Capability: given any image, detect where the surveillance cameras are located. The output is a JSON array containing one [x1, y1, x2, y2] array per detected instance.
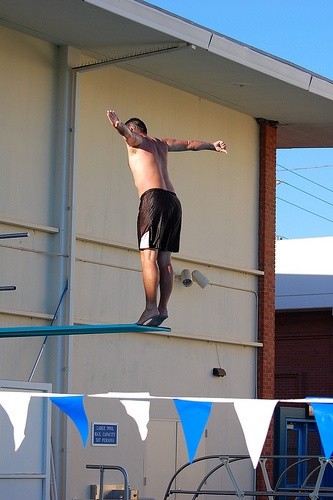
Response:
[[181, 268, 193, 288], [191, 269, 210, 289]]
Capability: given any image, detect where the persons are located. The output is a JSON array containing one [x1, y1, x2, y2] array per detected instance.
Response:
[[106, 110, 227, 327]]
